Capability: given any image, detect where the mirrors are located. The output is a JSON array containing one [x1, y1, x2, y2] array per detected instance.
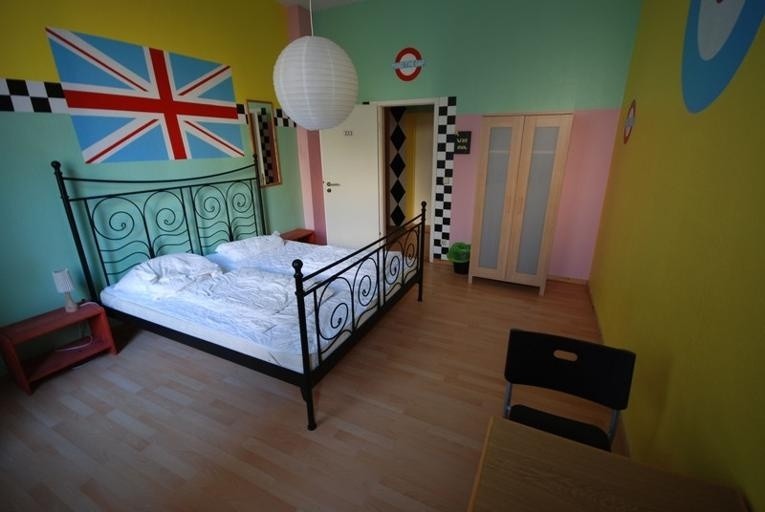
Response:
[[245, 98, 282, 188]]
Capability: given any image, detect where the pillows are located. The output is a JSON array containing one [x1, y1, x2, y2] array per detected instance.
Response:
[[215, 230, 285, 262], [113, 251, 225, 291]]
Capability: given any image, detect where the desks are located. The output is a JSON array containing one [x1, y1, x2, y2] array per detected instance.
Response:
[[280, 228, 315, 244], [2, 300, 120, 398], [464, 413, 751, 512]]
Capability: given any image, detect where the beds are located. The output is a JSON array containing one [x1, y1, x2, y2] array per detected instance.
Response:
[[51, 154, 427, 431]]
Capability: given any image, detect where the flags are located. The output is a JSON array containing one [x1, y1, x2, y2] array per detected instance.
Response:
[[42, 25, 246, 166]]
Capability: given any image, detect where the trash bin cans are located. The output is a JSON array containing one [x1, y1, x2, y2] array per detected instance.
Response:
[[452, 243, 470, 274]]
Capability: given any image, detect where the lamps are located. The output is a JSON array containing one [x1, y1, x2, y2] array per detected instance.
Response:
[[272, 1, 359, 132], [52, 268, 79, 312]]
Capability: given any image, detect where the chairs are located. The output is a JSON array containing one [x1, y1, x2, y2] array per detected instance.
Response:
[[502, 329, 636, 454]]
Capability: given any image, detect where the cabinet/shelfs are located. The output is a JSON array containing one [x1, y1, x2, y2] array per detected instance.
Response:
[[467, 110, 576, 298]]
